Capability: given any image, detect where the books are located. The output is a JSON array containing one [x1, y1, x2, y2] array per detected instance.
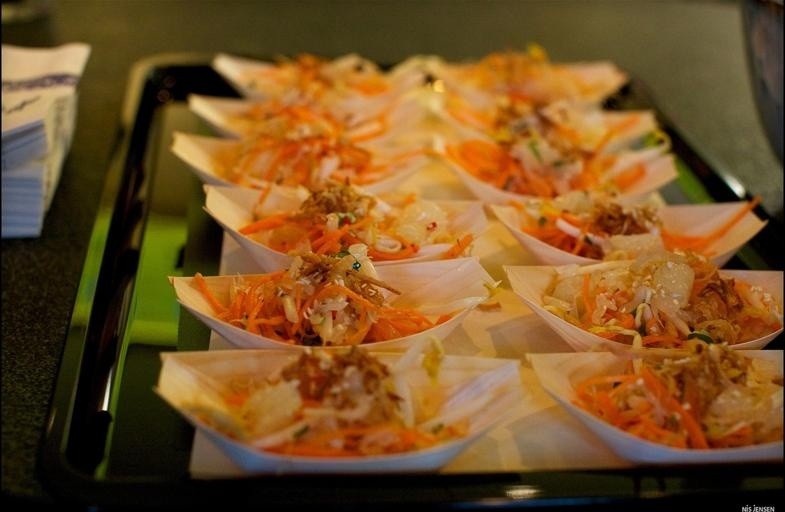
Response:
[[1, 41, 92, 239]]
